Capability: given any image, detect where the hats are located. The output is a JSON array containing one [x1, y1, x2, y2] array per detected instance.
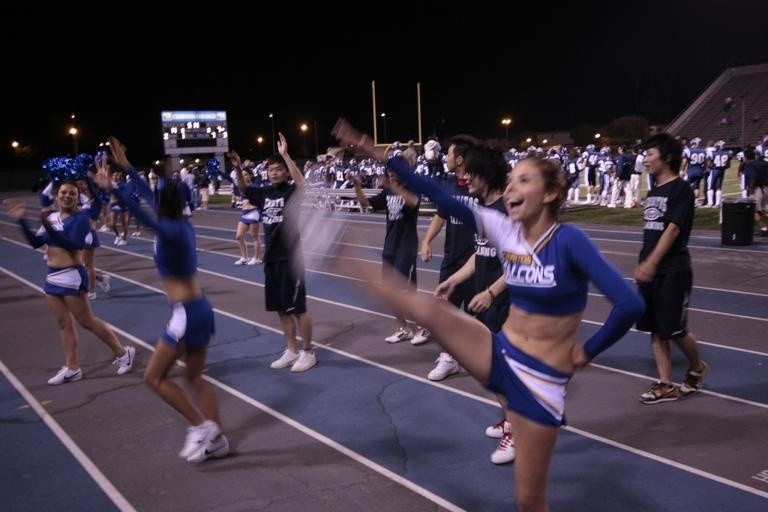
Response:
[[600, 146, 611, 155], [690, 137, 702, 145], [392, 141, 400, 150], [715, 141, 726, 149], [528, 145, 536, 155], [509, 148, 517, 157], [585, 144, 595, 151]]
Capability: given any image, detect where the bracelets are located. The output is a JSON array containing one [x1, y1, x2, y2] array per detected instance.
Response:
[[486, 286, 498, 300]]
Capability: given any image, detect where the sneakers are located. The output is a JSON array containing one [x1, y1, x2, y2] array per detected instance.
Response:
[[117, 240, 127, 246], [567, 197, 571, 201], [291, 351, 316, 372], [179, 420, 220, 458], [624, 203, 631, 208], [600, 199, 610, 206], [607, 203, 616, 208], [574, 199, 579, 205], [114, 237, 121, 245], [270, 349, 300, 369], [87, 292, 96, 301], [678, 360, 709, 399], [410, 326, 431, 344], [596, 198, 602, 204], [706, 201, 713, 208], [583, 200, 591, 205], [427, 352, 459, 380], [98, 275, 109, 293], [639, 381, 679, 404], [384, 328, 414, 344], [715, 203, 720, 208], [485, 420, 505, 438], [589, 200, 597, 204], [234, 257, 250, 265], [760, 230, 768, 237], [112, 346, 136, 375], [187, 435, 229, 463], [246, 257, 260, 265], [48, 366, 82, 385], [491, 421, 516, 464]]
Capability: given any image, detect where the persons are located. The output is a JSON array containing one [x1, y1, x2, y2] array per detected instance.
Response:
[[705, 141, 732, 209], [229, 131, 317, 374], [128, 213, 141, 238], [87, 138, 230, 464], [387, 140, 402, 158], [1, 182, 136, 385], [178, 163, 189, 181], [433, 143, 515, 465], [424, 134, 442, 178], [78, 176, 110, 302], [93, 152, 112, 234], [606, 145, 634, 210], [736, 153, 745, 202], [181, 169, 197, 211], [31, 174, 56, 261], [197, 174, 210, 209], [346, 148, 434, 347], [107, 173, 131, 247], [737, 148, 768, 236], [632, 132, 709, 405], [596, 146, 614, 207], [629, 147, 644, 206], [576, 143, 598, 205], [565, 151, 584, 204], [420, 134, 475, 381], [233, 168, 265, 267], [685, 139, 707, 208], [286, 118, 648, 512], [298, 155, 385, 188], [403, 136, 417, 170], [505, 146, 543, 167]]
